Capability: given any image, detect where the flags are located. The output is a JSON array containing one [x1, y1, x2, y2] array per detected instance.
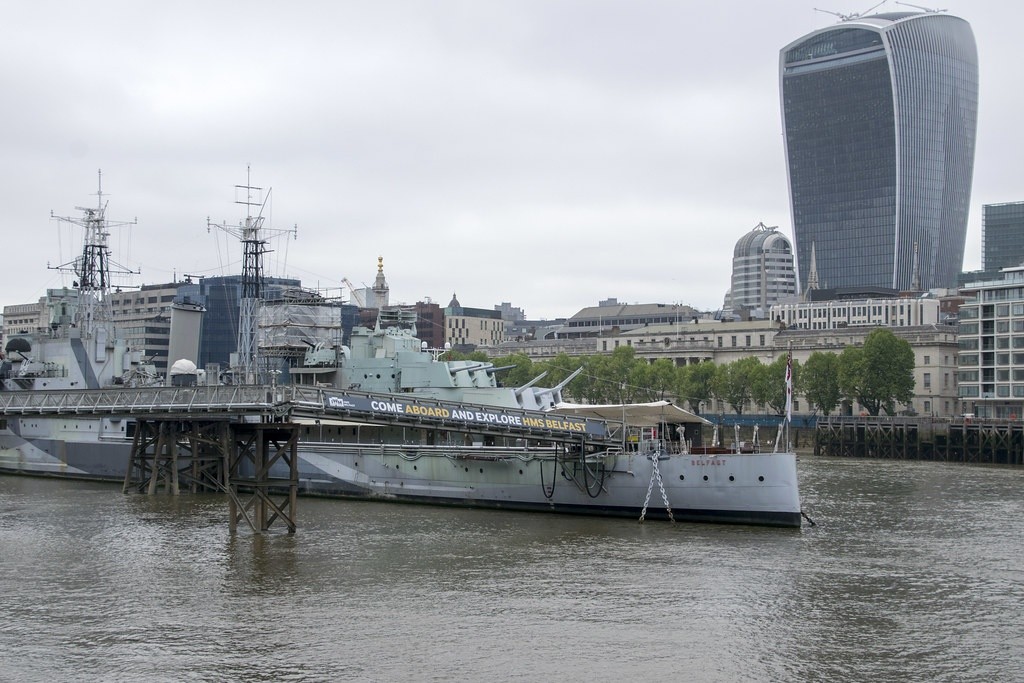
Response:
[[785, 350, 792, 423]]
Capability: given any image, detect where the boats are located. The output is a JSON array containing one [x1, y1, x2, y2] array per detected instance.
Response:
[[1, 156, 802, 530]]
